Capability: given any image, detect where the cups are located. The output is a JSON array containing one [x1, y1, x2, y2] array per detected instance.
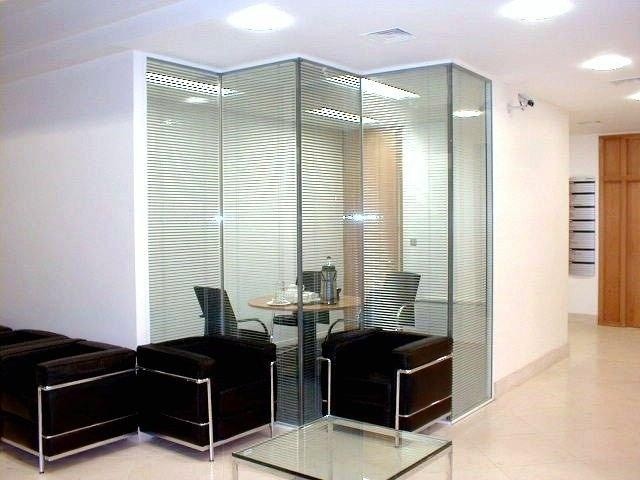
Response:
[[270, 280, 319, 304]]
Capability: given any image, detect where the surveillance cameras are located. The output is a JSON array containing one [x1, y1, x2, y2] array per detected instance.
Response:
[[517, 93, 534, 107]]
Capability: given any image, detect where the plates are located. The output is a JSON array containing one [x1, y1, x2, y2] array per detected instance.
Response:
[[266, 300, 292, 307]]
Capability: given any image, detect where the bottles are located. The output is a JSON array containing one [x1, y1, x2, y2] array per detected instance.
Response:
[[318, 266, 339, 304]]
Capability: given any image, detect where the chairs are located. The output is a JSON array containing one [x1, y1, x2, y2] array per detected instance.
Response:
[[193, 285, 270, 343], [137, 334, 278, 461], [1, 326, 137, 474], [327, 271, 421, 339], [319, 328, 452, 448]]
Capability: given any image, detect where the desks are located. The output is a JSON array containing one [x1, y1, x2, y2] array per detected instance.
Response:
[[247, 293, 363, 382]]
